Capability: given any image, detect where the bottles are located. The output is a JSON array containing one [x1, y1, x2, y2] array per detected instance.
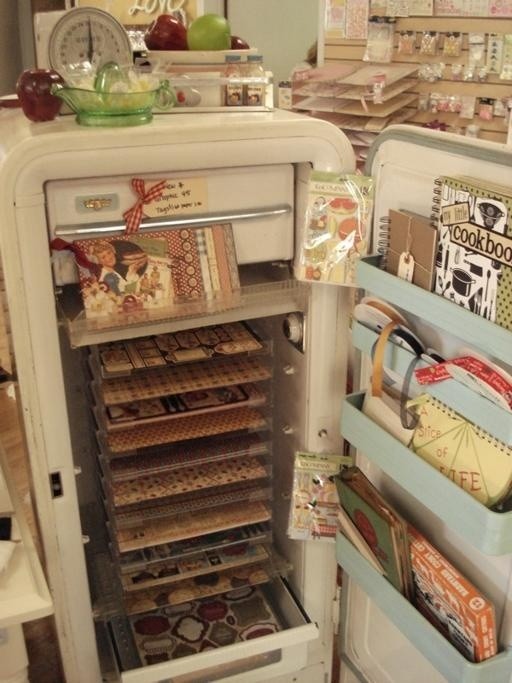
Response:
[[244, 55, 266, 107], [223, 55, 244, 106]]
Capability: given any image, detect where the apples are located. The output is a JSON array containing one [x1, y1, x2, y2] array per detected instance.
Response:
[[230, 36, 249, 50], [186, 14, 231, 50], [15, 69, 68, 123], [144, 13, 188, 51]]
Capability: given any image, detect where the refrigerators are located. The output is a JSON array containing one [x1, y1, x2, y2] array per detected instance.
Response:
[[0, 94, 512, 683]]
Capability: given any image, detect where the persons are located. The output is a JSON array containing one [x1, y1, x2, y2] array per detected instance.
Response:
[[89, 241, 162, 309]]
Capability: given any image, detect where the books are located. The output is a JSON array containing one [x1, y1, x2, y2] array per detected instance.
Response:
[[429, 174, 512, 331], [334, 464, 414, 605], [379, 207, 438, 294]]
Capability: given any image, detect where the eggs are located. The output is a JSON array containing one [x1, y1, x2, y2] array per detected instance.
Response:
[[175, 88, 203, 106]]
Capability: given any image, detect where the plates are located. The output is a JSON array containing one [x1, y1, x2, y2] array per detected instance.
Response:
[[146, 46, 259, 63]]
